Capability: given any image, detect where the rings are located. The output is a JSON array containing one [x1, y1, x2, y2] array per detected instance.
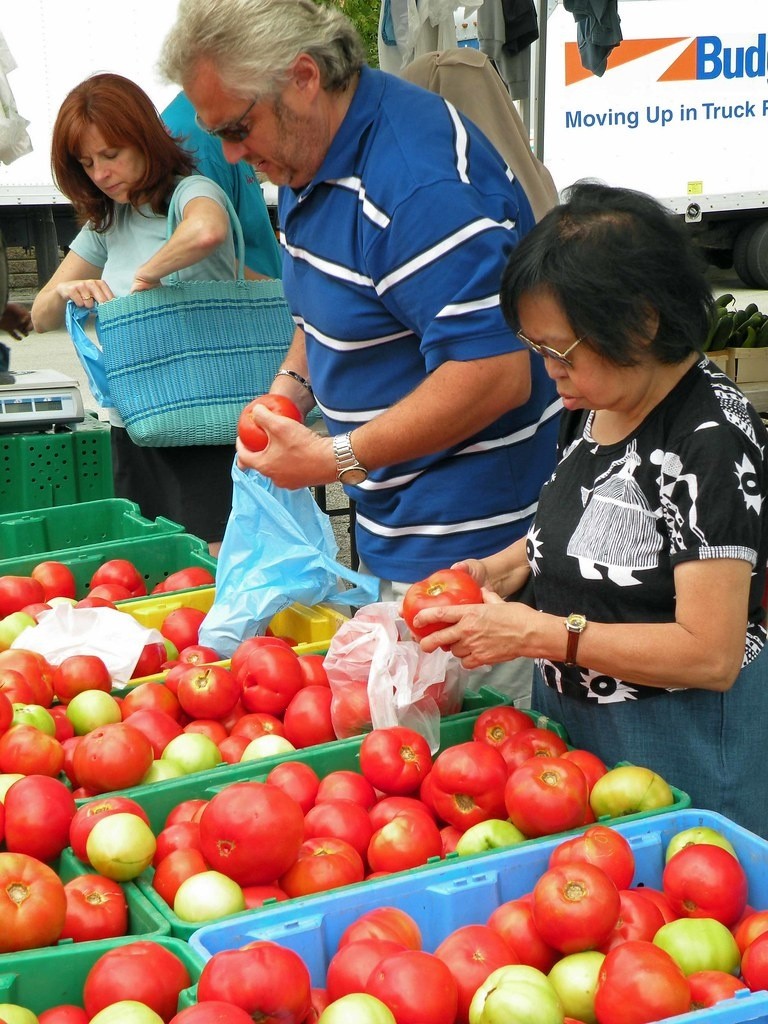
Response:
[[459, 659, 472, 671], [82, 295, 94, 301]]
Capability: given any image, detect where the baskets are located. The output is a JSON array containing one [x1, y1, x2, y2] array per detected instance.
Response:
[[0, 499, 768, 1023]]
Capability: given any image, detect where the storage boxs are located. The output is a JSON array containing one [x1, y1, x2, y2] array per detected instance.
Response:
[[0, 497, 768, 1024], [0, 409, 115, 514]]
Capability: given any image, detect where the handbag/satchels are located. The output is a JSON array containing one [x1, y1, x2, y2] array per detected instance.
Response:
[[324, 602, 491, 762], [198, 450, 379, 658], [97, 175, 323, 447]]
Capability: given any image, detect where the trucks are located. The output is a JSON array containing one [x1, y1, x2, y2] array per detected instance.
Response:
[[375, 0, 768, 291]]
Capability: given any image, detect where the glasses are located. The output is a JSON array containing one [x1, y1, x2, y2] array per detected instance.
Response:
[[513, 329, 593, 368], [196, 95, 259, 142]]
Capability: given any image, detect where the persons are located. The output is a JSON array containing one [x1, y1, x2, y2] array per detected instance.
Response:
[[399, 177, 767, 839], [0, 0, 566, 602]]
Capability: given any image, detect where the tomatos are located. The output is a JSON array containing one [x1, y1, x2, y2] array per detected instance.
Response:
[[0, 559, 768, 1024], [238, 394, 303, 452]]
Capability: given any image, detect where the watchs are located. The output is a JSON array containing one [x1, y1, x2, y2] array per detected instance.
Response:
[[333, 430, 368, 485], [563, 612, 587, 667]]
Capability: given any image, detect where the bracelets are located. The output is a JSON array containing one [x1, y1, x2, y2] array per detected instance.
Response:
[[274, 370, 317, 407]]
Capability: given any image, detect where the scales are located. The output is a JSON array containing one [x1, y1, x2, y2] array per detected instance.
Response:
[[0, 368, 86, 429]]
